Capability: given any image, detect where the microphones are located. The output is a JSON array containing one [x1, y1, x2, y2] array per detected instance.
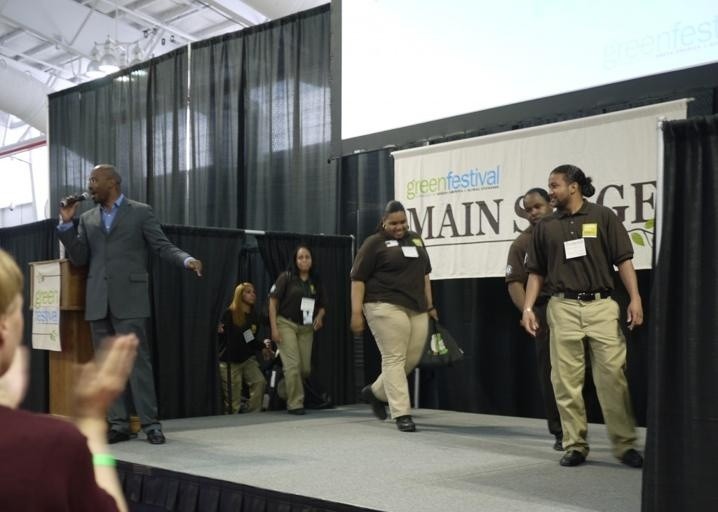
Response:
[[58, 192, 91, 207]]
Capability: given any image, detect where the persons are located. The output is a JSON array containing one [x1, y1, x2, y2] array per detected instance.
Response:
[[505, 188, 567, 451], [349, 200, 439, 432], [521, 164, 644, 469], [267, 243, 327, 415], [216, 282, 265, 413], [56, 164, 202, 445], [0, 249, 139, 511], [261, 338, 277, 360]]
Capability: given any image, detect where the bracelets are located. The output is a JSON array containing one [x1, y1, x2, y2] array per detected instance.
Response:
[[93, 452, 118, 469], [522, 307, 535, 313], [428, 306, 435, 313]]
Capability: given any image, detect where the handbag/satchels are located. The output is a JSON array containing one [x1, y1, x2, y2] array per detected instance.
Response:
[[426, 317, 464, 369]]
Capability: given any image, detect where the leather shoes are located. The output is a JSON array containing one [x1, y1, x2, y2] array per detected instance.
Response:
[[620, 448, 642, 468], [397, 416, 416, 432], [107, 430, 128, 443], [559, 451, 585, 466], [554, 288, 614, 302], [147, 429, 166, 444], [362, 385, 388, 419], [289, 407, 306, 415], [553, 437, 563, 451]]
